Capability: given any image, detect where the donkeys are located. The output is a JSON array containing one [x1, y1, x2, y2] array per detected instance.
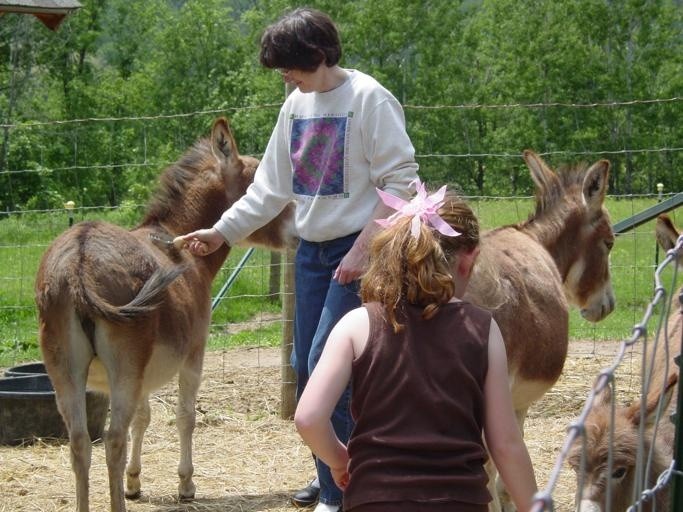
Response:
[[566, 212, 682, 512], [32, 118, 301, 512], [453, 149, 616, 512]]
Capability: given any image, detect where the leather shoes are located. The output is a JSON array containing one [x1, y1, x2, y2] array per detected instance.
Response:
[[291, 477, 320, 505]]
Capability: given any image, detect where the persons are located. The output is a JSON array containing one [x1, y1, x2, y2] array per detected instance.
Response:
[[183, 9, 420, 512], [293, 176, 538, 512]]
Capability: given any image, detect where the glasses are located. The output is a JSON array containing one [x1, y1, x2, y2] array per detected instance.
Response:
[[273, 68, 296, 79]]
